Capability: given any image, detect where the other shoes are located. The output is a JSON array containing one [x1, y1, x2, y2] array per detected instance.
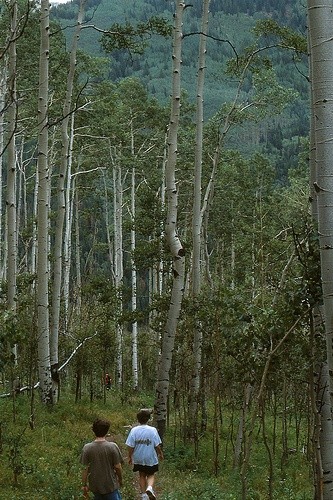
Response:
[[142, 496, 149, 500], [145, 488, 157, 500]]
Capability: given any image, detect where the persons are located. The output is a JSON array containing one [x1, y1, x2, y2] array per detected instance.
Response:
[[79, 420, 125, 500], [104, 374, 112, 390], [125, 409, 164, 500]]
